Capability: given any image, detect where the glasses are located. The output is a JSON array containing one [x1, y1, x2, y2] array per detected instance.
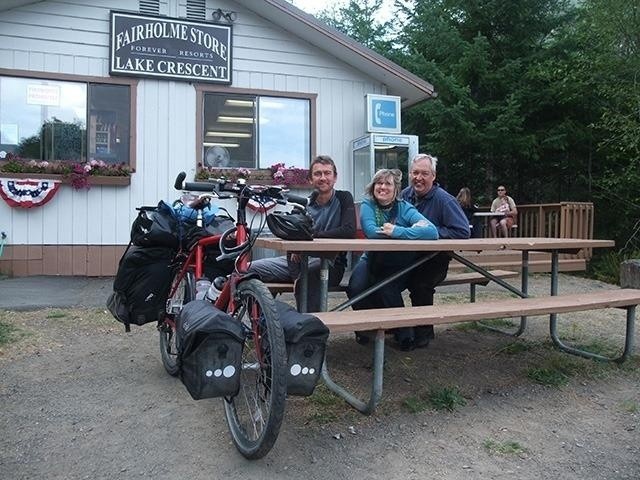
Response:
[[497, 189, 505, 191]]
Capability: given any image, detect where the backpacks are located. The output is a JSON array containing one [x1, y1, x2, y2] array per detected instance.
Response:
[[107, 207, 237, 332]]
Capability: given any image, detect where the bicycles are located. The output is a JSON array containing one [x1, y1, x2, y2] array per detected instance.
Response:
[[105, 171, 328, 460]]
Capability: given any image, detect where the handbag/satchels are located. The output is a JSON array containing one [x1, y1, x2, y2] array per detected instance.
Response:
[[175, 300, 245, 400], [261, 299, 330, 397]]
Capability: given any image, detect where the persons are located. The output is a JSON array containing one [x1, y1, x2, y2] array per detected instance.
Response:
[[347, 153, 469, 349], [455, 186, 481, 238], [490, 184, 519, 238], [246, 156, 356, 313], [358, 168, 438, 347]]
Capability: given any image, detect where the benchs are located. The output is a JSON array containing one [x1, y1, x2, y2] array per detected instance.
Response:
[[469, 212, 518, 238], [254, 268, 514, 337], [300, 285, 640, 406]]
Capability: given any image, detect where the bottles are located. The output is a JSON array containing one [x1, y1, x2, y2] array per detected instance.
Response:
[[201, 276, 227, 303]]
